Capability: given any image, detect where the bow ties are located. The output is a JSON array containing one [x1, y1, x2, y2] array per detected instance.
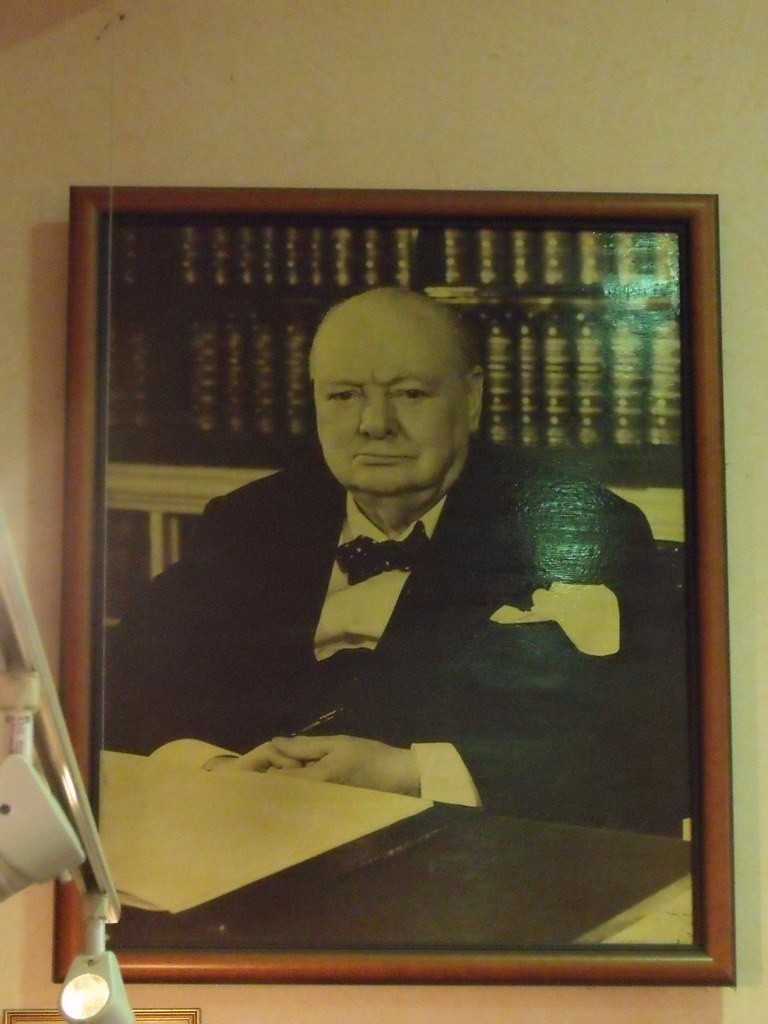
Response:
[[335, 519, 430, 586]]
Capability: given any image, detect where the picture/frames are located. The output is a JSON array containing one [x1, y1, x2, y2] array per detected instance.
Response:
[[47, 185, 739, 989]]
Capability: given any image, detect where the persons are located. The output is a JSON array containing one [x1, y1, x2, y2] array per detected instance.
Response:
[[102, 288, 693, 835]]
